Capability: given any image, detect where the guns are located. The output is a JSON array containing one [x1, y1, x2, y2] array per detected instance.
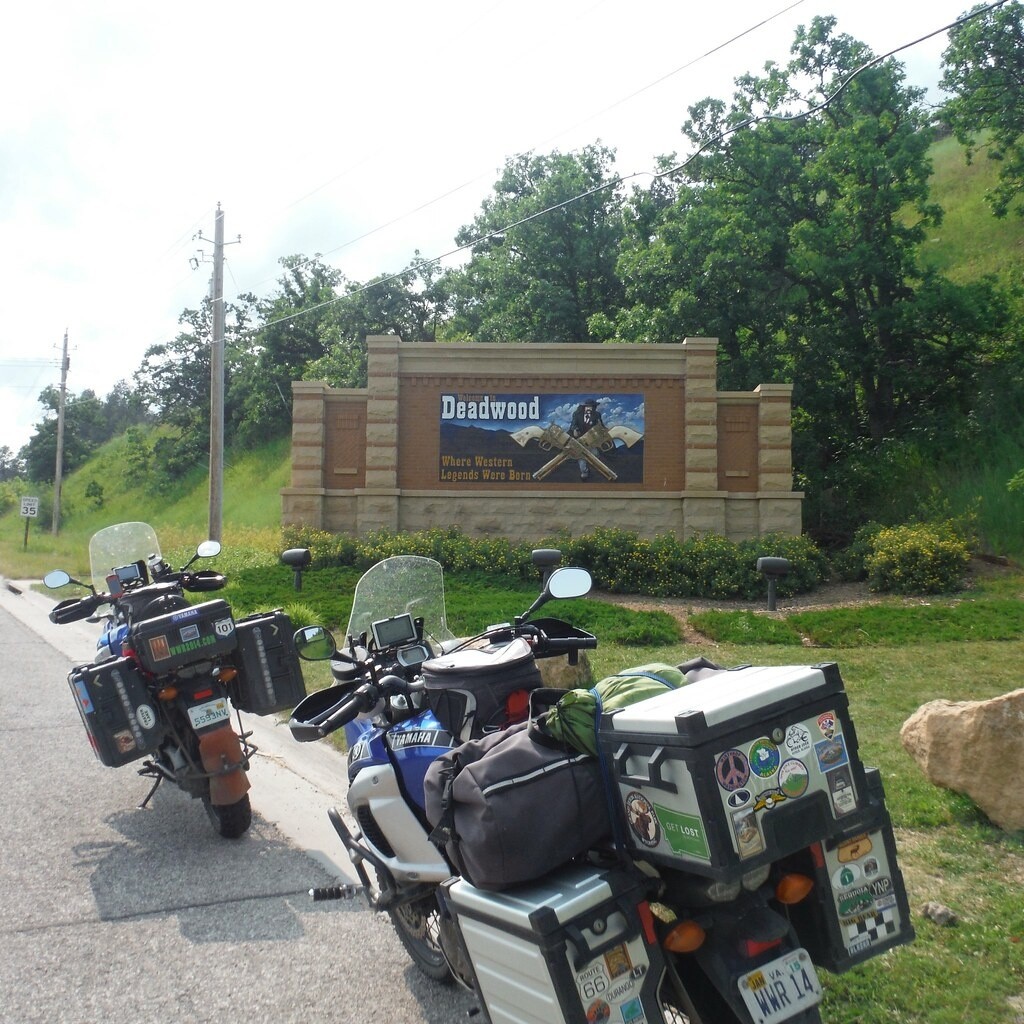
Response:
[[532, 425, 643, 481], [510, 424, 617, 481]]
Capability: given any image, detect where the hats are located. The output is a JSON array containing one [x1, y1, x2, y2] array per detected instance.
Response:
[[583, 398, 600, 407]]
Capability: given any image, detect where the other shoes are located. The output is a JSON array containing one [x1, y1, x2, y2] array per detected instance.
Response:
[[580, 472, 589, 479]]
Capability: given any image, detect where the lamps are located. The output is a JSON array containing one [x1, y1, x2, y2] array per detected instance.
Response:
[[757, 557, 789, 610], [282, 549, 311, 592], [532, 550, 561, 591]]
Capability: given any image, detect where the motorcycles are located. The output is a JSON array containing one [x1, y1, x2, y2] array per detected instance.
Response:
[[287, 555, 917, 1024], [41, 521, 310, 842]]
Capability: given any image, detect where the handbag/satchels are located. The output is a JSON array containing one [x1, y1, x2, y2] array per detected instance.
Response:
[[423, 655, 727, 890]]
